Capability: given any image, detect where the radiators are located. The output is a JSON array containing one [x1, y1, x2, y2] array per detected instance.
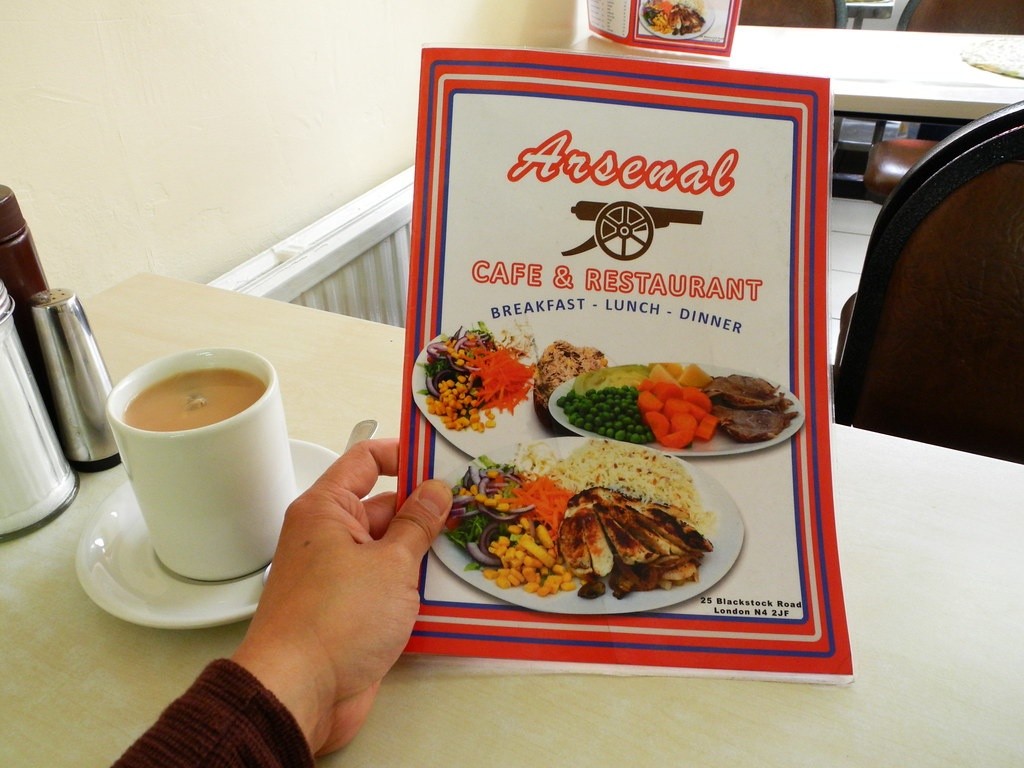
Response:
[[205, 163, 415, 327]]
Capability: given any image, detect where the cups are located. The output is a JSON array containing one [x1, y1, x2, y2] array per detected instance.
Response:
[[105, 348, 298, 581]]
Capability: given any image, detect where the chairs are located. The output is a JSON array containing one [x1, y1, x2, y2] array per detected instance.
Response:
[[736, 0, 1024, 464]]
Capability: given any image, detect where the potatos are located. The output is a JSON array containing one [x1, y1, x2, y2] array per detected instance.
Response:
[[647, 362, 714, 389]]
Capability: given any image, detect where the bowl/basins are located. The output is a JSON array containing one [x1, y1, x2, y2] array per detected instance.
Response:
[[533, 386, 581, 437]]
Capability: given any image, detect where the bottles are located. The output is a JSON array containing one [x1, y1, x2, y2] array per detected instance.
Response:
[[0, 183, 90, 437], [31, 288, 121, 473]]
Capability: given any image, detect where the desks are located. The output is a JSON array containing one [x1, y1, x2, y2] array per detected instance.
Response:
[[1, 273, 1024, 768], [568, 24, 1024, 160]]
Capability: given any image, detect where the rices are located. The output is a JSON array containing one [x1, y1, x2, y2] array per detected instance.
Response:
[[544, 439, 716, 537]]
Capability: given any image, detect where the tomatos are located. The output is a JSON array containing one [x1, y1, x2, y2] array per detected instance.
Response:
[[573, 365, 652, 395]]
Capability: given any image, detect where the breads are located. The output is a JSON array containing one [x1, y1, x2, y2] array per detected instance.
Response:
[[559, 486, 716, 580]]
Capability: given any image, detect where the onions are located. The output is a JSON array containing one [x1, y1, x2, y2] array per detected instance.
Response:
[[425, 326, 490, 398]]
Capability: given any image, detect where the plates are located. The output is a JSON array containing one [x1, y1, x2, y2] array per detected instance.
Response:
[[75, 440, 368, 630], [549, 363, 804, 455], [639, 0, 715, 39], [430, 437, 745, 614], [412, 319, 618, 460]]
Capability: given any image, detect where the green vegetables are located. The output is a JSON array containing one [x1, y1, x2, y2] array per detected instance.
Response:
[[444, 454, 520, 571]]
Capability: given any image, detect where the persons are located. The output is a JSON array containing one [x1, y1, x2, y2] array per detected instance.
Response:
[[109, 440, 454, 768]]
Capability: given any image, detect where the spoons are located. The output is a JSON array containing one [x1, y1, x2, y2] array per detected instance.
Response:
[[262, 421, 377, 585]]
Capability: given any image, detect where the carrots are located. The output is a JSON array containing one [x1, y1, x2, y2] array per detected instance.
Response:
[[636, 380, 718, 447], [500, 475, 575, 542], [463, 343, 539, 416]]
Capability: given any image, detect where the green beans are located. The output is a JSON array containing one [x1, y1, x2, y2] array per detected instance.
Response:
[[555, 383, 654, 443]]
